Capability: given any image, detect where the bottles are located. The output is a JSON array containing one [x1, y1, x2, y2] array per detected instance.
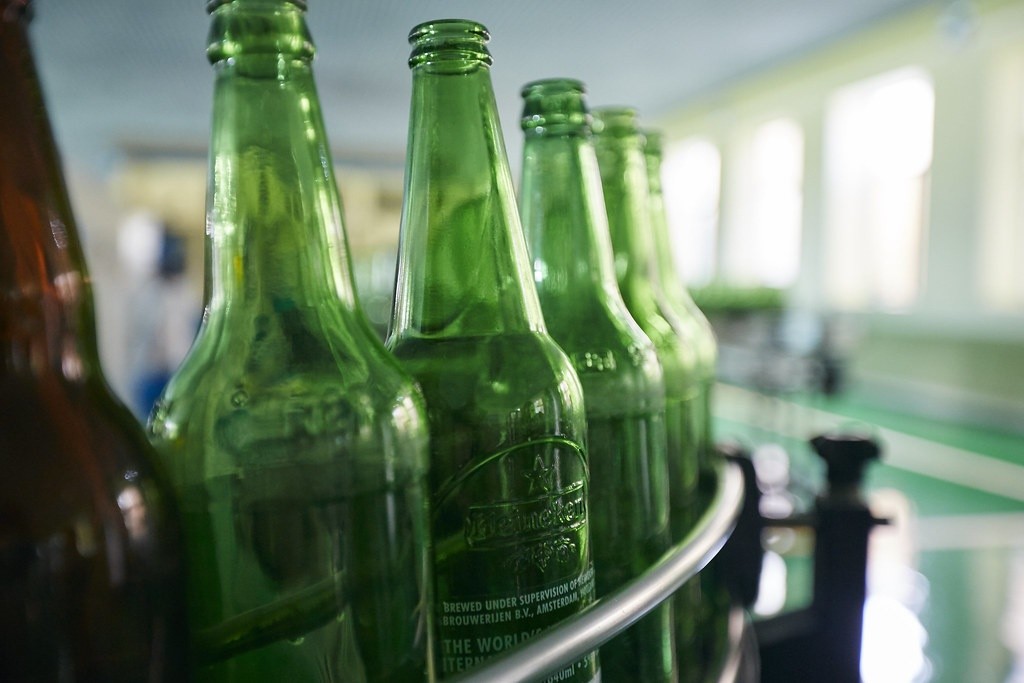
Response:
[[517, 78, 672, 683], [145, 1, 433, 683], [589, 105, 719, 683], [0, 0, 181, 683], [384, 19, 602, 683]]
